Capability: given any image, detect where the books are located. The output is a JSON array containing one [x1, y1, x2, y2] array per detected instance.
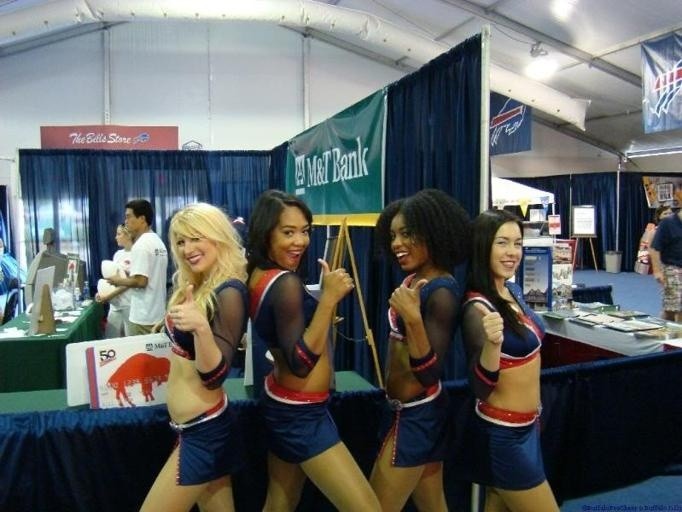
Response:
[[545, 298, 682, 344]]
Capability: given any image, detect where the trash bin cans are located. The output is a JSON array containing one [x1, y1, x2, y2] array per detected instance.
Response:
[[605, 250, 623, 273]]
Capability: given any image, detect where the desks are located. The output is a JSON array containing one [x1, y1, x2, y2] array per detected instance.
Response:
[[0, 371, 378, 415], [0, 297, 107, 393], [534, 301, 682, 369]]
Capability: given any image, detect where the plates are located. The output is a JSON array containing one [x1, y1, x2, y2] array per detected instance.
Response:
[[554, 302, 663, 332]]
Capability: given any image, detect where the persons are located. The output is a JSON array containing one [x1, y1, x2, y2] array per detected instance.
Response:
[[135, 200, 250, 512], [636, 201, 674, 277], [106, 197, 169, 335], [649, 181, 681, 325], [456, 208, 562, 512], [93, 221, 136, 340], [246, 184, 385, 512], [366, 185, 478, 511]]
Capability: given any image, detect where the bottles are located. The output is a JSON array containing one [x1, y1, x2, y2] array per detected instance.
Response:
[[83, 280, 90, 302], [73, 288, 82, 311]]
